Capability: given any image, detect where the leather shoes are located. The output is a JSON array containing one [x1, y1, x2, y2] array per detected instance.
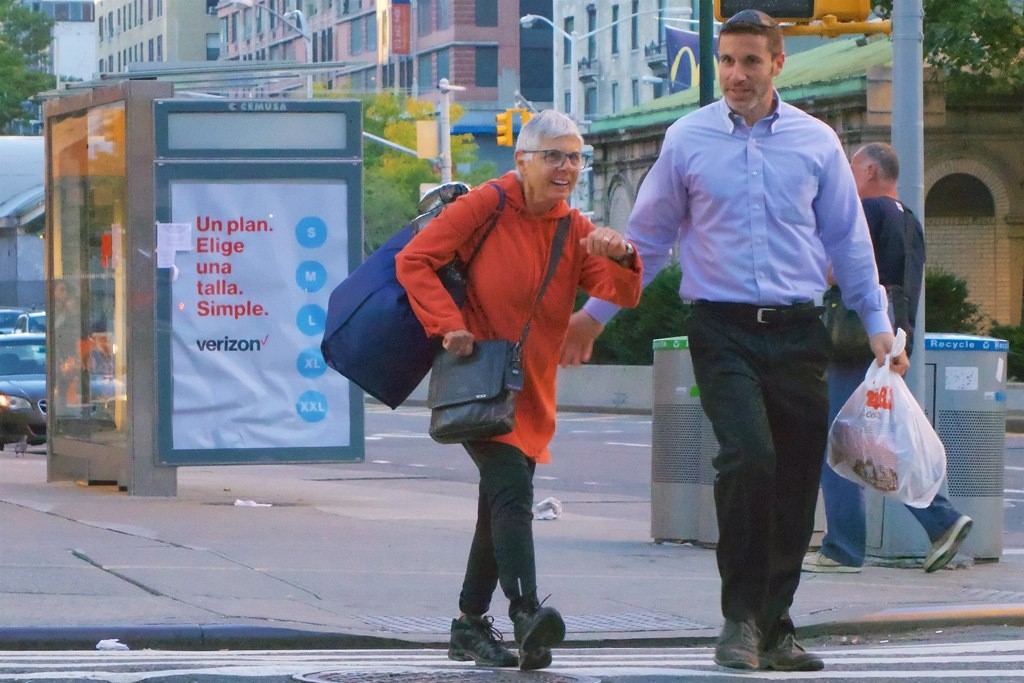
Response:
[[758, 629, 825, 672], [714, 614, 760, 671], [691, 298, 826, 327]]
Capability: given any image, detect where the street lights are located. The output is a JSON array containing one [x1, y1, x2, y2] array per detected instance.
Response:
[[232, 0, 312, 101], [520, 5, 695, 221]]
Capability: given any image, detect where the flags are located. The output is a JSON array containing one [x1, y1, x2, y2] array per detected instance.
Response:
[[665, 25, 721, 92]]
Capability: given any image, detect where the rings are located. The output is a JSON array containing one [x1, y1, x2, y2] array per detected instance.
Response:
[[604, 237, 609, 242]]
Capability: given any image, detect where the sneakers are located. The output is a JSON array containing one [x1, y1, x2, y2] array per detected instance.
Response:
[[448, 614, 520, 668], [513, 594, 566, 670], [801, 551, 862, 574], [922, 514, 974, 573]]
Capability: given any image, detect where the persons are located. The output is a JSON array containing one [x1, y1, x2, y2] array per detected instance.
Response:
[[394, 107, 643, 671], [53, 280, 81, 409], [801, 142, 974, 574], [558, 10, 910, 670]]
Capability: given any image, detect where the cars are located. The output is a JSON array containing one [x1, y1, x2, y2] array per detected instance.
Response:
[[0, 305, 120, 452]]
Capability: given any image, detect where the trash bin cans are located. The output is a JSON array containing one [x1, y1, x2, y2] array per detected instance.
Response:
[[861, 332, 1009, 563], [650, 338, 719, 549]]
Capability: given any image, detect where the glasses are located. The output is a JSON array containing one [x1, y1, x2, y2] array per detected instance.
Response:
[[523, 149, 592, 171]]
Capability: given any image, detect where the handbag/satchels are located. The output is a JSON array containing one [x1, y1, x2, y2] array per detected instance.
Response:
[[825, 327, 946, 509], [320, 180, 509, 409], [823, 287, 916, 367], [426, 336, 521, 443]]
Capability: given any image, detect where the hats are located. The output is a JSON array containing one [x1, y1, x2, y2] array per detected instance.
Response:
[[725, 9, 778, 28]]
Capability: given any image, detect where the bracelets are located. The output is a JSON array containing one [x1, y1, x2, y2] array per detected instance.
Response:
[[625, 242, 634, 255]]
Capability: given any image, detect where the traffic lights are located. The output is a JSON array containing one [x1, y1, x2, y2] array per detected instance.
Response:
[[714, 0, 872, 23]]
